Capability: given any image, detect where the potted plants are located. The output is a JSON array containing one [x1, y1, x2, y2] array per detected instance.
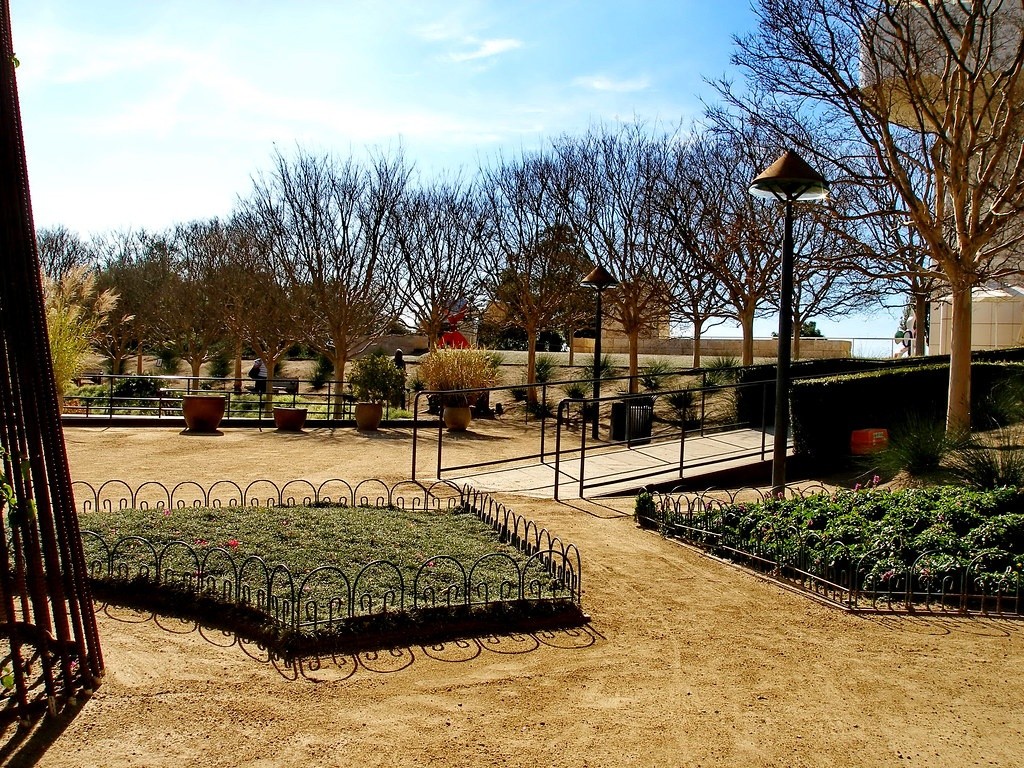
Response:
[[346, 353, 407, 431], [418, 338, 501, 433]]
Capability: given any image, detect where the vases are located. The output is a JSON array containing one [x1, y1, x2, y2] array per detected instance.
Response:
[[181, 394, 227, 434], [273, 407, 308, 432]]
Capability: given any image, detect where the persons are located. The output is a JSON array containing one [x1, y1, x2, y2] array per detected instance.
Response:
[[253, 351, 269, 392], [389, 349, 406, 410]]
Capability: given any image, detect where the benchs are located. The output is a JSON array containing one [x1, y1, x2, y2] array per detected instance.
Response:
[[71, 367, 104, 386], [271, 376, 300, 394]]
